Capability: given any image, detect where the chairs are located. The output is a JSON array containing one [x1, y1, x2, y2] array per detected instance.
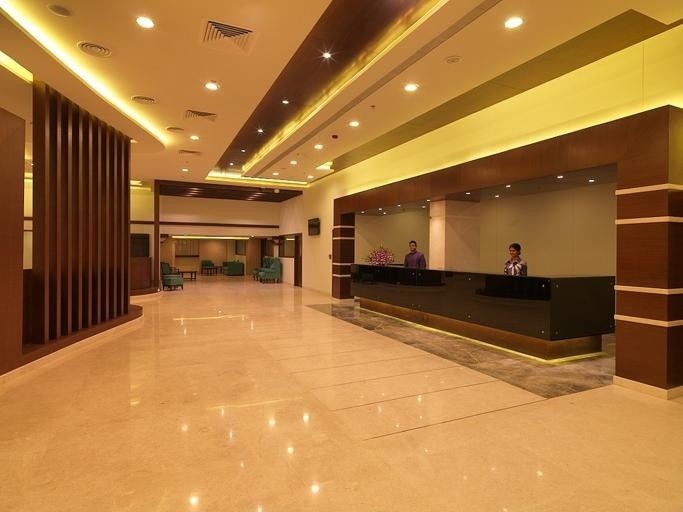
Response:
[[160, 255, 282, 291]]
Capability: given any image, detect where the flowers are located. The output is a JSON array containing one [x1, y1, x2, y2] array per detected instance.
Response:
[[360, 240, 395, 266]]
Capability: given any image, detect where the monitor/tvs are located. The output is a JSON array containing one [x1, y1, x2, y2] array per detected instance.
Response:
[[307, 218, 320, 236]]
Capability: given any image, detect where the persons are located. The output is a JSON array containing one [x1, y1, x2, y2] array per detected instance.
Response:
[[404, 240, 426, 269], [504, 242, 527, 277]]
